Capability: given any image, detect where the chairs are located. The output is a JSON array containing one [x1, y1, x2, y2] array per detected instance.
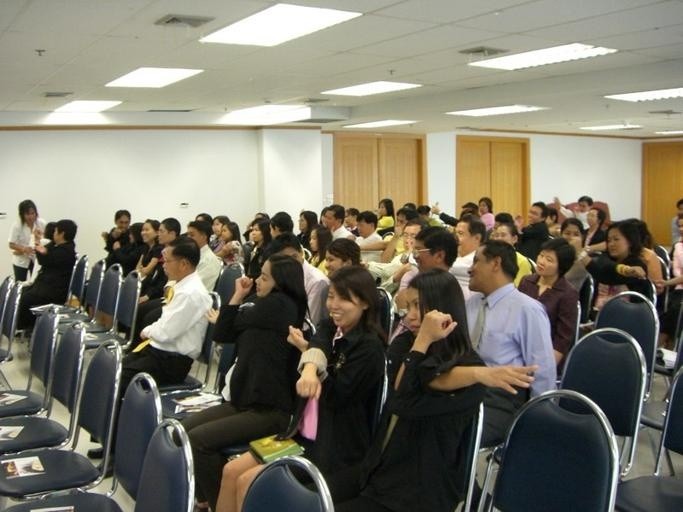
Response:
[[0, 248, 317, 512], [241, 455, 335, 511], [377, 241, 683, 512]]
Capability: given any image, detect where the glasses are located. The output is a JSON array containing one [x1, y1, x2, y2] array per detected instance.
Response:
[[410, 247, 431, 257]]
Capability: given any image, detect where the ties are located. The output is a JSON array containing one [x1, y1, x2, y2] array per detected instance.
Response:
[[131, 287, 173, 353], [471, 298, 488, 349]]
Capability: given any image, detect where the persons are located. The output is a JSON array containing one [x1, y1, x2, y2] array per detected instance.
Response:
[[459, 240, 556, 510], [78, 238, 210, 458], [213, 266, 387, 512], [170, 254, 306, 504], [306, 270, 538, 511]]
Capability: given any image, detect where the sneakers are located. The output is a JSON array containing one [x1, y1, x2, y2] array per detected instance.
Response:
[[95, 462, 114, 477], [87, 446, 104, 458]]
